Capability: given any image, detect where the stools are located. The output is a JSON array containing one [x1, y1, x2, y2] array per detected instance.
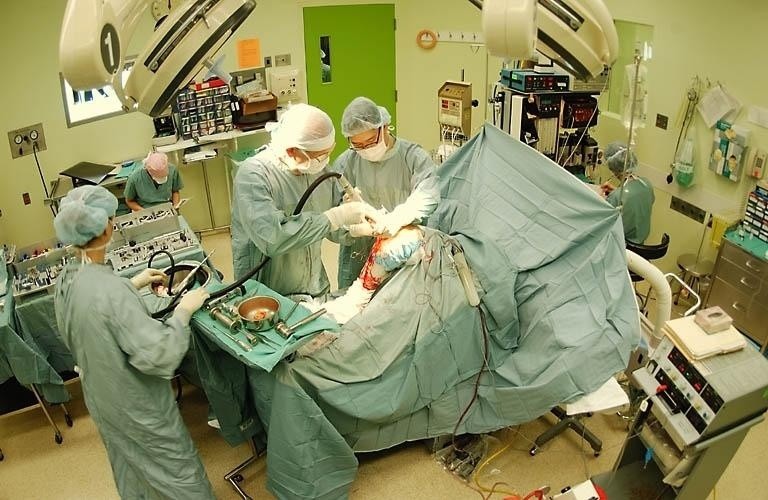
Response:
[[530, 375, 630, 458], [669, 252, 715, 305]]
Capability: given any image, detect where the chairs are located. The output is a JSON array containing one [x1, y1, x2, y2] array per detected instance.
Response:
[[624, 233, 672, 294]]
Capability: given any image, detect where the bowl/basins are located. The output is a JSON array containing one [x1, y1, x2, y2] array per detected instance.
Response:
[[237, 294, 281, 331]]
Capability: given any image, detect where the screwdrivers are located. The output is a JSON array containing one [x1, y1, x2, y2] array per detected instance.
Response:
[[212, 324, 253, 354]]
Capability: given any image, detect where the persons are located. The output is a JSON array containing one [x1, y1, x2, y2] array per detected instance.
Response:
[[124, 152, 183, 213], [219, 105, 362, 299], [601, 143, 655, 243], [52, 187, 214, 500], [298, 220, 425, 326], [329, 97, 438, 290]]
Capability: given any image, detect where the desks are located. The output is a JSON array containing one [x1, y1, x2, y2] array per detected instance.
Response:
[[44, 156, 145, 217], [151, 124, 270, 244], [0, 249, 72, 460], [13, 215, 223, 428]]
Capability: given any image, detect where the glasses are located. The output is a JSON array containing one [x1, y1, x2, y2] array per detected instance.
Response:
[[307, 143, 338, 164], [346, 128, 382, 151]]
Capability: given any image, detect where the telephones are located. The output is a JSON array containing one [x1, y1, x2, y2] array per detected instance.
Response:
[[745, 148, 766, 179]]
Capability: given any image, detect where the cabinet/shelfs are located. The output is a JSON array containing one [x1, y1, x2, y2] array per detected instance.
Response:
[[702, 238, 768, 355]]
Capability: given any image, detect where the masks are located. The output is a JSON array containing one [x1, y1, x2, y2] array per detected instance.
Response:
[[148, 171, 168, 185], [350, 125, 391, 162], [284, 147, 330, 175]]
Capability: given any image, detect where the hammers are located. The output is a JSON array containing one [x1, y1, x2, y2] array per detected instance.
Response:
[[275, 308, 327, 339]]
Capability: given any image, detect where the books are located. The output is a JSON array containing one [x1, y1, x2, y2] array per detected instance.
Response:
[[664, 317, 746, 362]]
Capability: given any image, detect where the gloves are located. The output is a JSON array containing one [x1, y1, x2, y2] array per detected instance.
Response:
[[129, 267, 168, 290], [180, 286, 211, 315], [323, 186, 409, 239]]
